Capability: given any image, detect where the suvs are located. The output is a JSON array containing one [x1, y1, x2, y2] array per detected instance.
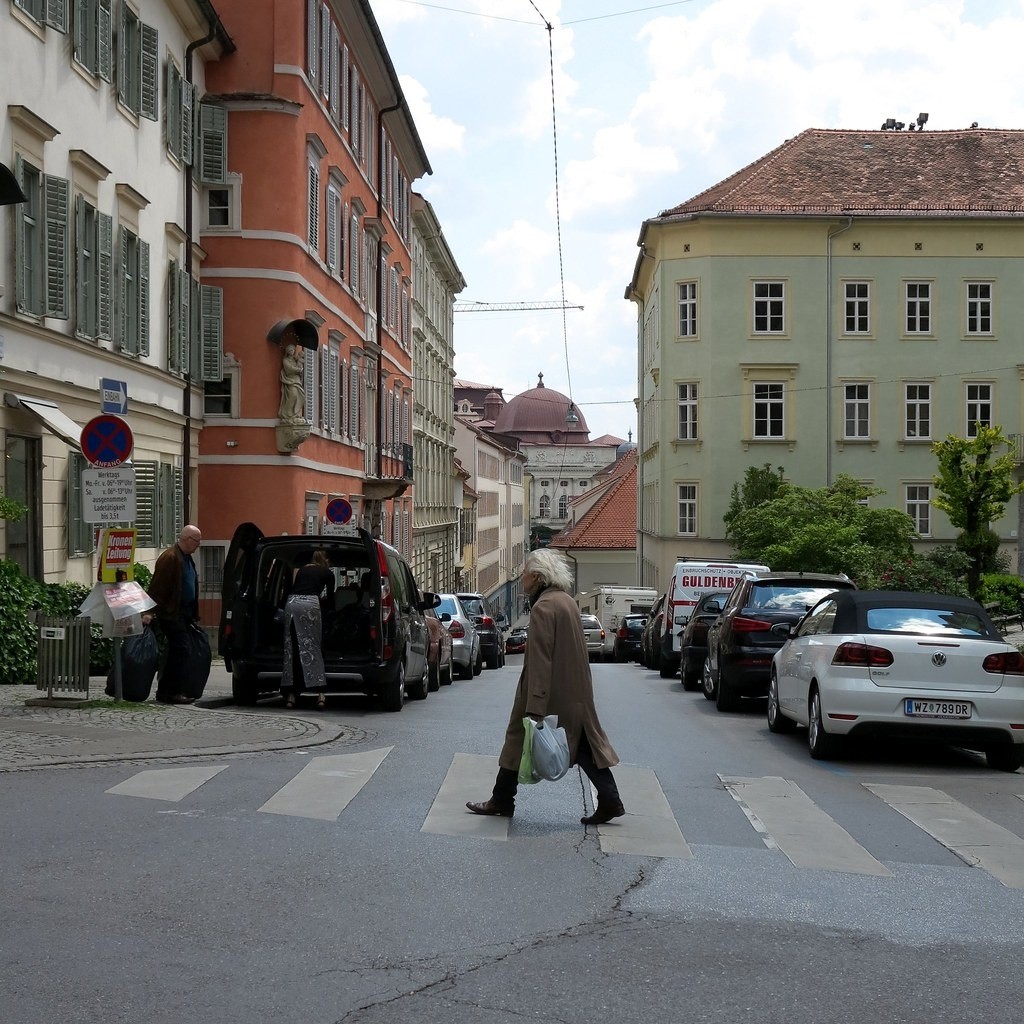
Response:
[[456, 591, 511, 670], [700, 568, 860, 712]]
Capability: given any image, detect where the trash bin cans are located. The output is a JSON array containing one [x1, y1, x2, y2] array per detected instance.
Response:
[[25, 616, 91, 708]]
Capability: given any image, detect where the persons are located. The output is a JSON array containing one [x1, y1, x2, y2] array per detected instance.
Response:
[[281, 550, 335, 711], [141, 524, 203, 703], [464, 550, 626, 825]]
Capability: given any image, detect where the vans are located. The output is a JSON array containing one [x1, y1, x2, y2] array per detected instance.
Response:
[[659, 554, 771, 678], [217, 523, 444, 712]]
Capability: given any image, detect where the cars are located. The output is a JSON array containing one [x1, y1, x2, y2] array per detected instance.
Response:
[[418, 589, 455, 692], [578, 613, 606, 662], [504, 635, 526, 654], [764, 589, 1024, 773], [434, 594, 484, 680], [510, 625, 528, 640], [610, 613, 649, 663], [639, 593, 667, 670], [674, 587, 735, 692]]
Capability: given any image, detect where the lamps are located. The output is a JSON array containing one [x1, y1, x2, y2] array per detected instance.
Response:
[[565, 403, 579, 427]]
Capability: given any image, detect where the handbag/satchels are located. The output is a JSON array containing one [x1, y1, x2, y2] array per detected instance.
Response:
[[176, 619, 212, 699], [518, 715, 569, 785], [105, 624, 157, 702]]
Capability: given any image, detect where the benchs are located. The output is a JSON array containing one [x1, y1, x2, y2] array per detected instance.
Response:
[[984, 600, 1024, 636]]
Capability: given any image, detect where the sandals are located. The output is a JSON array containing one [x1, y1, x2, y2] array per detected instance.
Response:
[[285, 701, 296, 709], [316, 701, 326, 710]]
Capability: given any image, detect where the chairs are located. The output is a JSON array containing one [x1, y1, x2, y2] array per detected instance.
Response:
[[335, 587, 358, 611], [361, 572, 373, 591]]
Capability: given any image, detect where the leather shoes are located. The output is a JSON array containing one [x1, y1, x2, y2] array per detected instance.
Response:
[[466, 798, 515, 818], [580, 804, 625, 825], [156, 690, 195, 704]]
